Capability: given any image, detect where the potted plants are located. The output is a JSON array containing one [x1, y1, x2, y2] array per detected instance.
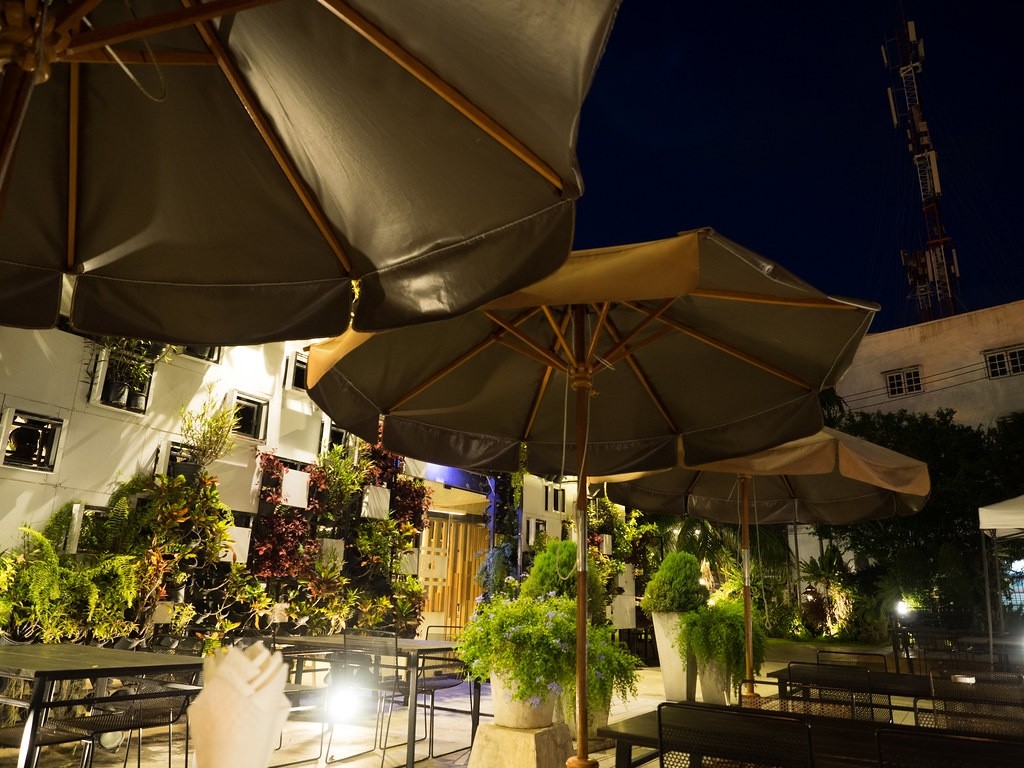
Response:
[[661, 603, 768, 706], [78, 334, 188, 412], [516, 538, 616, 739], [639, 548, 710, 702], [0, 382, 436, 680]]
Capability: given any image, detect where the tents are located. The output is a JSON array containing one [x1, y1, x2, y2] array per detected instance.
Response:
[[977, 489, 1024, 677]]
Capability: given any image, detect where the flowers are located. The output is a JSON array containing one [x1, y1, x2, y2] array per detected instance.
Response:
[[450, 576, 648, 725]]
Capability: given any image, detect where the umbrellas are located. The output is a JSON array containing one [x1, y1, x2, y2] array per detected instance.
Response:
[[2, 1, 622, 349], [302, 228, 883, 759], [588, 425, 932, 696]]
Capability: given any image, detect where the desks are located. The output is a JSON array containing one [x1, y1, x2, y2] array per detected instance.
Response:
[[767, 664, 1024, 737], [0, 643, 207, 768], [244, 635, 482, 768], [595, 699, 1024, 768], [887, 623, 1024, 687]]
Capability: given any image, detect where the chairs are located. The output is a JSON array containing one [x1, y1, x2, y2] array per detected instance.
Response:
[[43, 633, 207, 768], [913, 694, 1024, 739], [812, 650, 892, 723], [737, 679, 857, 720], [928, 665, 1024, 736], [375, 626, 473, 759], [325, 628, 433, 768], [657, 702, 818, 768], [231, 628, 329, 768], [787, 662, 893, 723], [873, 728, 1024, 768], [0, 723, 96, 768]]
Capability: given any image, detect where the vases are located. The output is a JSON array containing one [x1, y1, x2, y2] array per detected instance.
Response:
[[188, 347, 212, 358], [488, 667, 557, 731]]
